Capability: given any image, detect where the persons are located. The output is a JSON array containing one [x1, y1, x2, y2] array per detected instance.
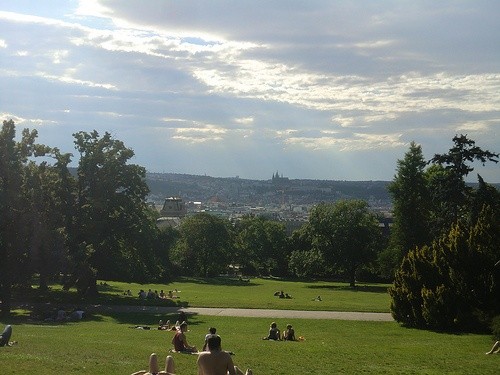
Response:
[[197, 335, 252, 375], [138, 289, 173, 301], [203, 327, 222, 351], [229, 280, 234, 286], [131, 353, 175, 375], [315, 296, 322, 301], [268, 322, 281, 341], [240, 277, 243, 281], [170, 321, 191, 332], [274, 291, 292, 299], [128, 290, 132, 296], [282, 324, 295, 341], [57, 307, 66, 321], [248, 278, 251, 282], [100, 282, 110, 288], [172, 324, 198, 353], [0, 325, 13, 347], [158, 323, 169, 330], [485, 341, 500, 354], [75, 308, 87, 320]]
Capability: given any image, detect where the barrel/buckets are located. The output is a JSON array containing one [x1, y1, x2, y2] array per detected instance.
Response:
[[76, 311, 84, 320]]
